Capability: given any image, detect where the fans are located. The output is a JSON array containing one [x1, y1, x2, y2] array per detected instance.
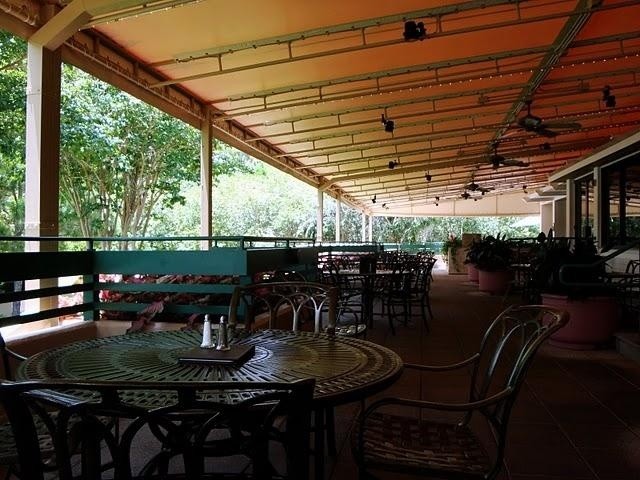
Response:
[[446, 96, 583, 201]]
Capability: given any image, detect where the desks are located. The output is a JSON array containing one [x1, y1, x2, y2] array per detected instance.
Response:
[[22, 323, 398, 418]]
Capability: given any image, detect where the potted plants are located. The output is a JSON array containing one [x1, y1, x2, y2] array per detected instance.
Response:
[[529, 234, 620, 350], [474, 235, 512, 294], [463, 241, 479, 281]]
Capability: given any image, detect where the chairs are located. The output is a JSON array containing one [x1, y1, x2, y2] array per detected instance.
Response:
[[351, 305, 568, 479], [317, 236, 438, 334], [229, 280, 333, 331], [5, 375, 315, 480]]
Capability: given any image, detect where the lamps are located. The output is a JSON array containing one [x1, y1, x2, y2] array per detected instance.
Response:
[[402, 20, 426, 42], [603, 88, 615, 108], [389, 160, 397, 168], [381, 113, 394, 132]]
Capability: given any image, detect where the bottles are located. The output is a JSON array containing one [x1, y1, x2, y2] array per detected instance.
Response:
[[216, 315, 231, 351], [200, 314, 215, 349]]
